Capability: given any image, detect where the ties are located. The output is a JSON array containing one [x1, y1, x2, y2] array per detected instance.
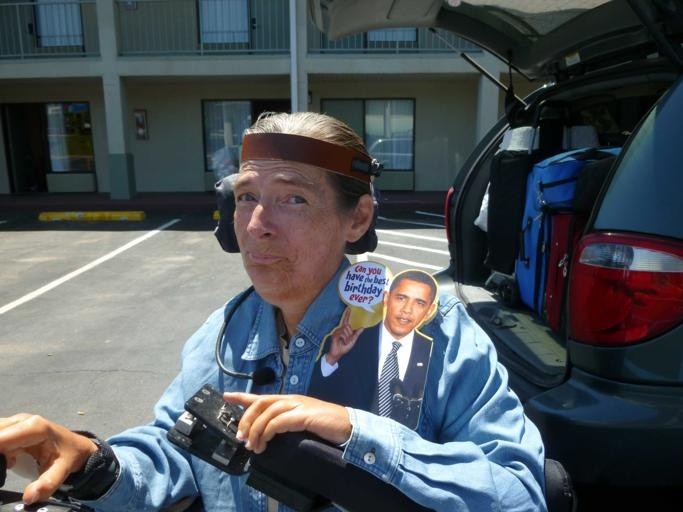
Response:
[[379, 340, 403, 420]]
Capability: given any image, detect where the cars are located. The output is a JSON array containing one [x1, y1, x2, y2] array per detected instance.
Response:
[[305, 0, 679, 507], [368, 137, 413, 169]]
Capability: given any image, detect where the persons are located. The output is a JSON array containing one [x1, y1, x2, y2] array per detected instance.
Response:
[[0, 113, 551, 511], [307, 271, 443, 433]]
